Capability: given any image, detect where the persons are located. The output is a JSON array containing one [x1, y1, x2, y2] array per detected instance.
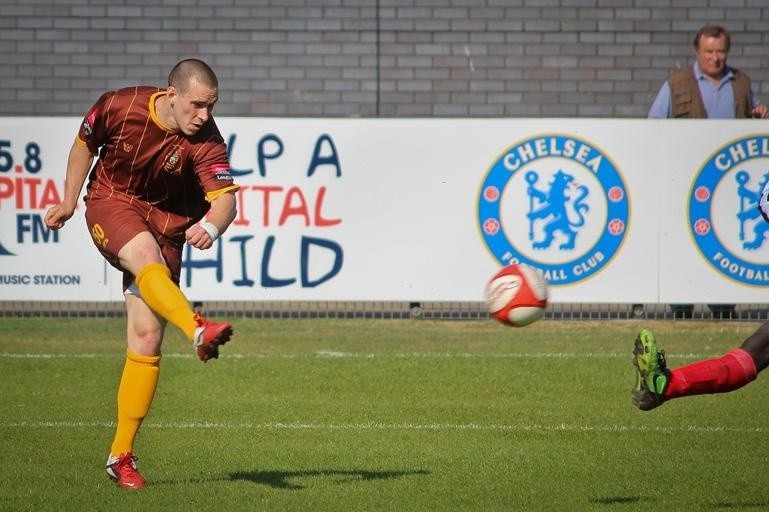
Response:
[[632, 320, 769, 412], [44, 59, 241, 494], [647, 24, 768, 120]]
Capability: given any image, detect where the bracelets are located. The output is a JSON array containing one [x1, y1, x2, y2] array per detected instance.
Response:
[[201, 221, 219, 240]]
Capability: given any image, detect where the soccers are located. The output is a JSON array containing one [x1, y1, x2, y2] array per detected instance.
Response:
[[485, 264, 548, 328]]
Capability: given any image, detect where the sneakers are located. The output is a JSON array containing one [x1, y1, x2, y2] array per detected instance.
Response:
[[106, 451, 148, 491], [192, 311, 234, 362], [630, 327, 672, 411]]
